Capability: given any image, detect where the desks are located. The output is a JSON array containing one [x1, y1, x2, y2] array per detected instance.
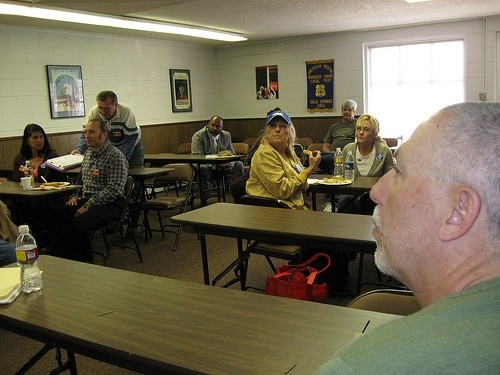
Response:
[[170, 202, 377, 287], [144, 154, 246, 207], [0, 182, 82, 237], [306, 175, 380, 211], [4, 167, 175, 243], [0, 255, 407, 375]]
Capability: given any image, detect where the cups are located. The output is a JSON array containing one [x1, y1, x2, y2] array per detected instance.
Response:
[[20, 177, 31, 190]]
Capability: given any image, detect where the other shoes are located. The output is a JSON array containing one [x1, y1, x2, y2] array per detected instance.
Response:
[[126, 224, 138, 236], [104, 223, 118, 233]]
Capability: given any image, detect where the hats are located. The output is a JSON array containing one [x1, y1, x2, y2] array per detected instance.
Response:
[[266, 110, 291, 124]]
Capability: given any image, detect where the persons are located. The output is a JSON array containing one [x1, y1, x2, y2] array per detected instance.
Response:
[[70, 90, 144, 240], [228, 108, 281, 204], [11, 123, 68, 255], [336, 114, 394, 283], [315, 101, 500, 375], [321, 99, 357, 213], [0, 201, 20, 268], [245, 110, 355, 299], [52, 119, 129, 263], [187, 116, 244, 207]]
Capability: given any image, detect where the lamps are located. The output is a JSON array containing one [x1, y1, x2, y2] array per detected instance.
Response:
[[0, 0, 248, 42]]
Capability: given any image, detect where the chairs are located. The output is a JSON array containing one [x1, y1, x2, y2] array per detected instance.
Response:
[[1, 138, 420, 316]]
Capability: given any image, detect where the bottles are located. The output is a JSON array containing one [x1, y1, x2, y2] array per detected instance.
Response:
[[16, 224, 42, 294], [334, 148, 343, 178], [24, 161, 35, 188], [345, 150, 355, 181]]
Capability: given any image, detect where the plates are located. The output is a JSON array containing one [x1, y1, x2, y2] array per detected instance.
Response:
[[316, 179, 353, 185], [216, 155, 235, 158], [40, 182, 70, 188]]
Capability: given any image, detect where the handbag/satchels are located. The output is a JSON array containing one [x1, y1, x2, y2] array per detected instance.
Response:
[[265, 252, 331, 302]]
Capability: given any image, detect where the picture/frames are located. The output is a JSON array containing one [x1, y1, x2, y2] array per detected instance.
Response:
[[46, 65, 86, 119]]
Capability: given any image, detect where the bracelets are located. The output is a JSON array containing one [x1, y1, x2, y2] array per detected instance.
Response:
[[84, 202, 90, 209]]
